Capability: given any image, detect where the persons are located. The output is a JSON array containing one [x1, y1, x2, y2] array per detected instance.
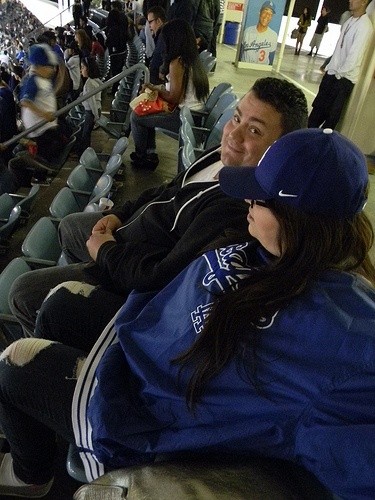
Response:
[[238, 0, 278, 65], [0, 129, 375, 500], [7, 77, 309, 339], [1, 0, 375, 173]]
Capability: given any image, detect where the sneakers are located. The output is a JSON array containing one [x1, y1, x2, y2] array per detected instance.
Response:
[[31, 176, 52, 186]]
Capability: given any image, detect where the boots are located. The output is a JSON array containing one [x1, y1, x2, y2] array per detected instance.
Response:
[[294, 49, 301, 56]]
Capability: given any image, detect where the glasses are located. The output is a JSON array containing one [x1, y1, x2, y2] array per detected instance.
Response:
[[148, 18, 158, 24], [251, 200, 272, 208]]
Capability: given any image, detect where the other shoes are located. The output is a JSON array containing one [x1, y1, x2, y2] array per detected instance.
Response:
[[0, 452, 54, 498], [70, 152, 79, 157], [307, 50, 313, 56], [130, 152, 159, 172]]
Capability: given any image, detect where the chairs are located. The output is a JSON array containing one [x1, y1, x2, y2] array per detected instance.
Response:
[[0, 8, 237, 348]]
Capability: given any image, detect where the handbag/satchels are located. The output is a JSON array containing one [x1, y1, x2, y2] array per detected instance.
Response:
[[129, 84, 177, 117], [291, 29, 299, 39]]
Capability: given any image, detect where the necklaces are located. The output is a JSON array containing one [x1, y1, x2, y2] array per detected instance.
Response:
[[340, 11, 367, 48]]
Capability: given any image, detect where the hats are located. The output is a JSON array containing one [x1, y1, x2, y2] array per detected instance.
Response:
[[261, 1, 276, 14], [219, 128, 368, 217], [28, 43, 59, 66]]
